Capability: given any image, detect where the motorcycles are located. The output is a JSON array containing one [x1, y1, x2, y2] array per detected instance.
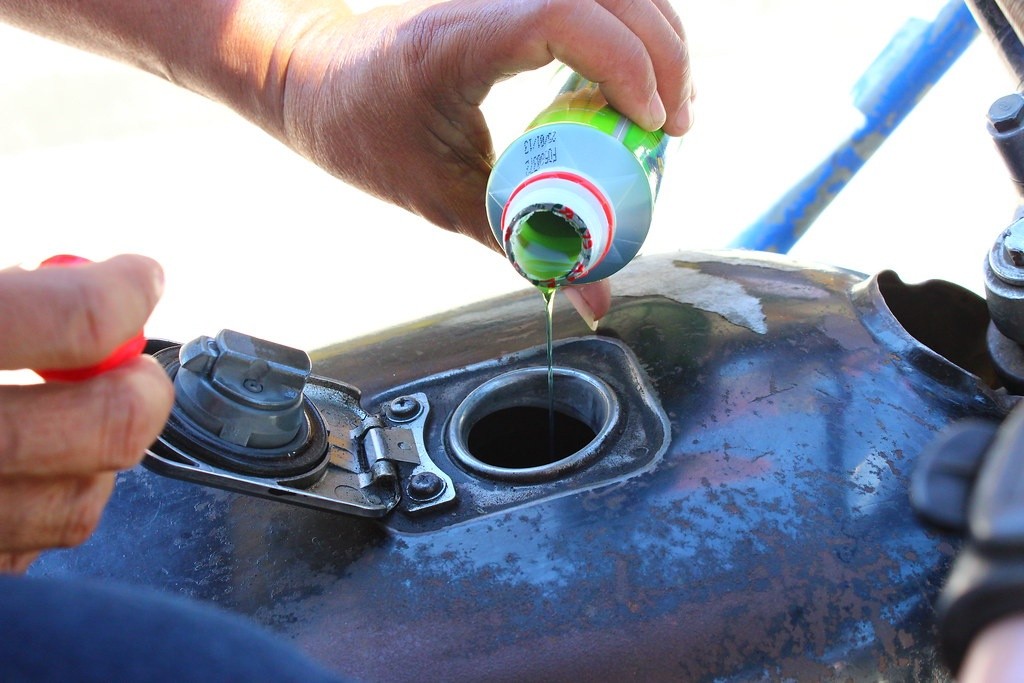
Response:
[[0, 1, 1024, 683]]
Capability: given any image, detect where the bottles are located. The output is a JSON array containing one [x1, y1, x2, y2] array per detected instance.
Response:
[[486, 70, 672, 287]]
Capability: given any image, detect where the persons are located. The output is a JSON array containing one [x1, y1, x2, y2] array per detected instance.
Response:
[[0, 0, 694, 576]]
